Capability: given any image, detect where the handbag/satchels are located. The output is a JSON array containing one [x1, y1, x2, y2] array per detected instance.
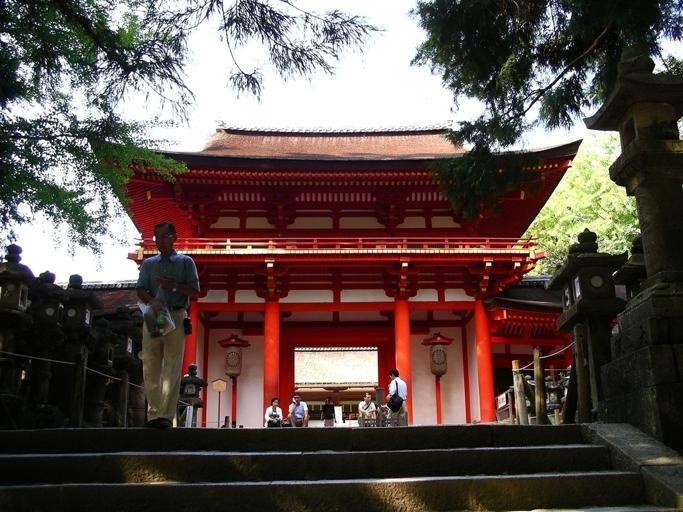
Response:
[[387, 393, 403, 413]]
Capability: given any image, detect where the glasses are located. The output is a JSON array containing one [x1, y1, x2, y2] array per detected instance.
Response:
[[153, 234, 173, 241]]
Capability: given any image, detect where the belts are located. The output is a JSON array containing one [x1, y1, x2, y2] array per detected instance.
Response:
[[168, 306, 184, 312]]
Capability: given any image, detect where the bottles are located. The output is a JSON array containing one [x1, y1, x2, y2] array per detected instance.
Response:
[[157, 308, 167, 333]]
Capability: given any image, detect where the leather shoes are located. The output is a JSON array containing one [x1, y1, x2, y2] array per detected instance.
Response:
[[146, 417, 173, 429]]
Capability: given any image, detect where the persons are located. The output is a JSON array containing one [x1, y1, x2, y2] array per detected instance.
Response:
[[287, 394, 310, 427], [358, 391, 377, 427], [385, 369, 407, 426], [134, 221, 200, 429], [321, 398, 336, 427], [265, 399, 283, 428]]
